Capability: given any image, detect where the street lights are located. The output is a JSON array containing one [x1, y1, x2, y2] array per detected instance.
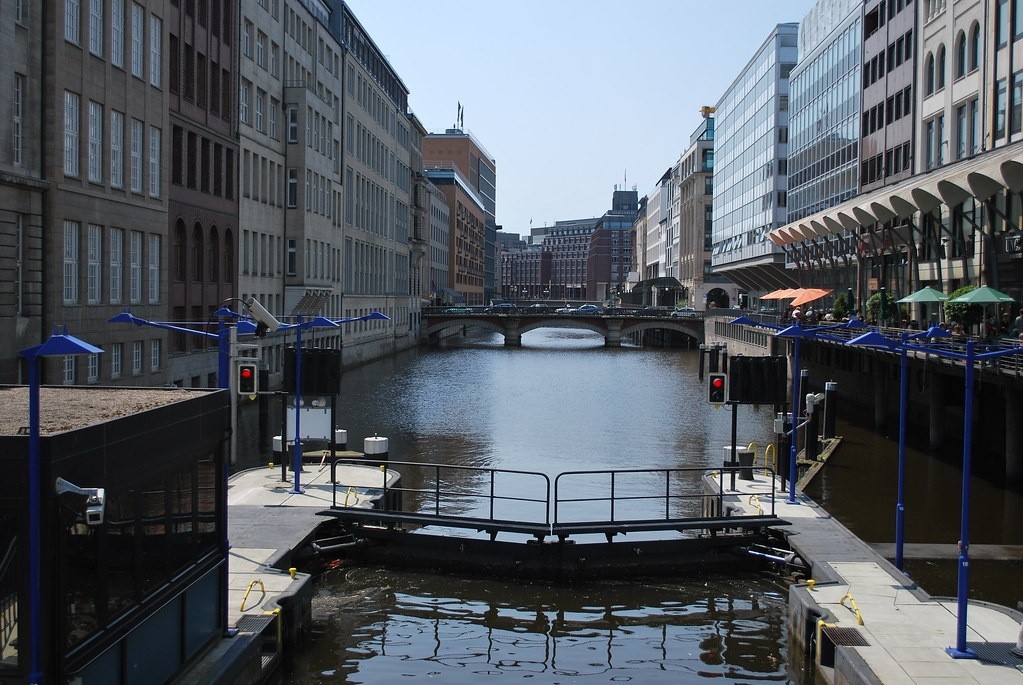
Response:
[[106, 312, 344, 548], [212, 305, 392, 494], [844, 330, 1023, 659], [17, 321, 107, 685], [728, 315, 871, 501], [772, 326, 953, 574]]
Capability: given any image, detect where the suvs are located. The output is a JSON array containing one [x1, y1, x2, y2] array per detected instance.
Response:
[[526, 303, 550, 314], [484, 303, 517, 315]]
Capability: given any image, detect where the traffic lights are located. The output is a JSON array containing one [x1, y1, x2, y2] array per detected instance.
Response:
[[237, 363, 257, 395], [708, 372, 728, 404]]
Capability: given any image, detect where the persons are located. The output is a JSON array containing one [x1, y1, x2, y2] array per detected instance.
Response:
[[985, 309, 1023, 348], [740, 302, 745, 309], [784, 305, 837, 321], [842, 309, 863, 322], [907, 319, 966, 350], [709, 301, 716, 310]]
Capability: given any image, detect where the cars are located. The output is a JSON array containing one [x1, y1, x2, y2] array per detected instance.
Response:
[[670, 307, 698, 319], [632, 305, 668, 317], [444, 305, 474, 315], [555, 303, 600, 316]]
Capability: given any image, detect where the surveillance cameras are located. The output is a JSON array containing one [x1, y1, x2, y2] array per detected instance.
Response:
[[86, 488, 106, 526], [243, 297, 280, 332]]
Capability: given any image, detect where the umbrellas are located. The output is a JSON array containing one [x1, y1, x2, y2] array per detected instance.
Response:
[[758, 287, 806, 300], [893, 286, 948, 330], [790, 287, 835, 319], [948, 285, 1014, 337]]
